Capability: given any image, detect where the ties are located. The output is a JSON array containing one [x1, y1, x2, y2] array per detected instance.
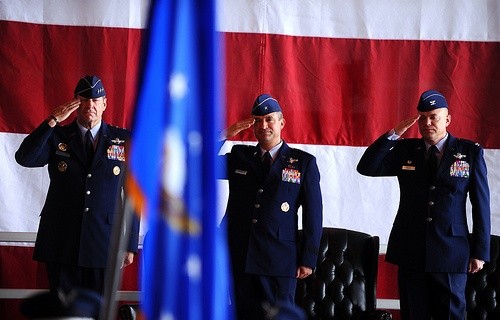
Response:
[[427, 144, 440, 170], [260, 151, 272, 172], [84, 129, 94, 159]]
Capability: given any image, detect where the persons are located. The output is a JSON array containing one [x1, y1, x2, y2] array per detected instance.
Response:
[[217, 93, 322, 297], [355, 90, 491, 319], [17, 74, 140, 291]]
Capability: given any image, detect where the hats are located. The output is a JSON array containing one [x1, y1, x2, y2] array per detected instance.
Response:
[[251, 93, 281, 116], [75, 74, 105, 99], [416, 89, 449, 112]]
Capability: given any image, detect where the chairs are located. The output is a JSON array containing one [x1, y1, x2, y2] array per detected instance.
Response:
[[465, 233, 500, 320], [294, 228, 391, 320]]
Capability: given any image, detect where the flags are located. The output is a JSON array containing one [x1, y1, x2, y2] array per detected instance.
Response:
[[128, 1, 235, 320]]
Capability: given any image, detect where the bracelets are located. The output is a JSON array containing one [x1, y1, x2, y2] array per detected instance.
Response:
[[50, 114, 59, 124]]
